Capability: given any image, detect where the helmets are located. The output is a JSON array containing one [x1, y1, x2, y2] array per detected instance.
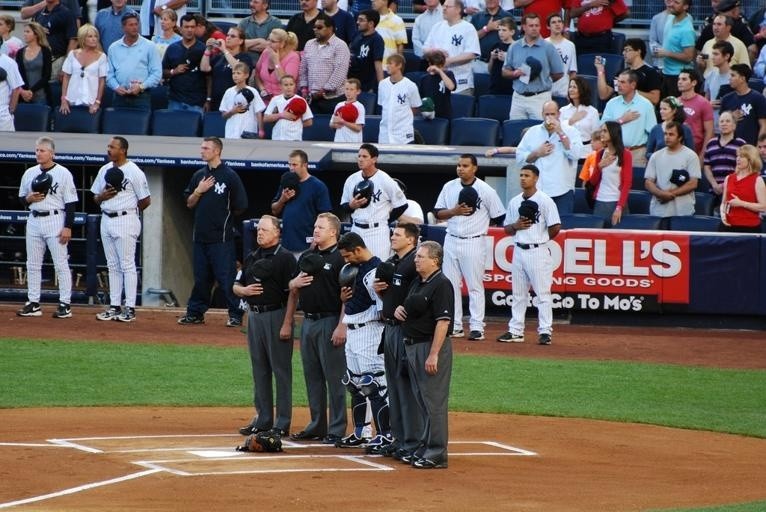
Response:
[[353, 180, 374, 208], [32, 173, 52, 196]]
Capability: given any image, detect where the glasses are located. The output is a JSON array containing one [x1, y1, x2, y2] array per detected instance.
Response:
[[81, 65, 85, 78], [626, 48, 631, 52]]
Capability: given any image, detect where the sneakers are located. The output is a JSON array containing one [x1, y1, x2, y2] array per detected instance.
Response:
[[17, 302, 42, 317], [240, 424, 448, 468], [449, 329, 552, 345], [96, 306, 136, 322], [226, 317, 243, 327], [53, 303, 72, 318], [178, 313, 205, 324]]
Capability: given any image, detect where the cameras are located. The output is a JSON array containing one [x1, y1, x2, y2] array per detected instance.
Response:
[[213, 40, 221, 48], [598, 58, 607, 65]]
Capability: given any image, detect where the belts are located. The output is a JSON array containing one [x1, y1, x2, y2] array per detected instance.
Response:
[[104, 210, 126, 217], [348, 320, 381, 329], [402, 333, 449, 345], [451, 234, 480, 239], [384, 318, 399, 327], [517, 243, 538, 249], [33, 210, 58, 217], [352, 219, 378, 228], [524, 90, 543, 96]]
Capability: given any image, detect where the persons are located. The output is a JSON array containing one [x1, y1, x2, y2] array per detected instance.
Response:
[[273, 143, 562, 345], [0, 0, 765, 145], [287, 212, 346, 443], [394, 240, 454, 470], [375, 222, 423, 461], [517, 69, 766, 226], [91, 137, 151, 323], [19, 135, 78, 322], [232, 214, 299, 440], [178, 137, 246, 327], [333, 230, 395, 453]]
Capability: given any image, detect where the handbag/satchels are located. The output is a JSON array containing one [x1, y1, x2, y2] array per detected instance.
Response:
[[720, 202, 731, 227], [585, 180, 595, 209]]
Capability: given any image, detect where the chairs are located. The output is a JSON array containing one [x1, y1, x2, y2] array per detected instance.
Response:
[[0, 0, 766, 235]]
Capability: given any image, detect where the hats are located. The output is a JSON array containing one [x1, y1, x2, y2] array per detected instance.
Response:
[[246, 253, 429, 321], [526, 57, 542, 81], [717, 0, 742, 11], [281, 172, 299, 199], [518, 201, 538, 224], [459, 187, 478, 216], [670, 169, 689, 186], [419, 98, 436, 120], [105, 167, 123, 191], [234, 89, 358, 122]]
